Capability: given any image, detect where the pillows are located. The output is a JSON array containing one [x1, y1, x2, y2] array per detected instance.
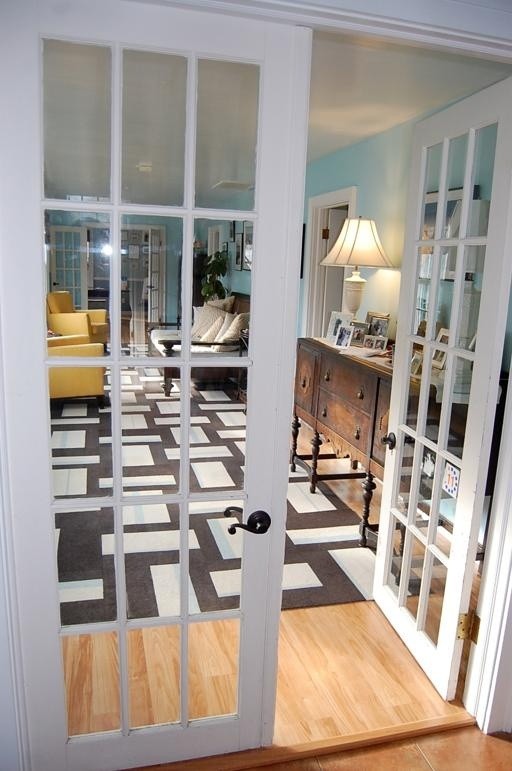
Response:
[[192, 296, 250, 353]]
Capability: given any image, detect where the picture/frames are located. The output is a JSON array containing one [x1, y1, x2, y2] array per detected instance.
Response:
[[121, 231, 149, 266], [220, 220, 252, 272], [416, 183, 481, 280], [326, 310, 451, 375]]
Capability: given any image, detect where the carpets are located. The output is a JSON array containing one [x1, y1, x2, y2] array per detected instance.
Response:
[[52, 339, 439, 628]]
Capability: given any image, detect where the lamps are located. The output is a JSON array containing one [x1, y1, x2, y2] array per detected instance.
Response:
[[319, 215, 398, 320]]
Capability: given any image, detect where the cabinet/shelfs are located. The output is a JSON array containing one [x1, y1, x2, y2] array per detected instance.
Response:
[[289, 337, 494, 560], [236, 329, 250, 418]]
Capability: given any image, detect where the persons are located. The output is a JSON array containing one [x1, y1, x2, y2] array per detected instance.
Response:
[[341, 332, 350, 345], [366, 339, 371, 347], [356, 330, 362, 339], [375, 341, 382, 349]]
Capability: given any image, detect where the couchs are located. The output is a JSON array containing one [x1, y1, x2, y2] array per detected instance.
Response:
[[146, 292, 250, 398]]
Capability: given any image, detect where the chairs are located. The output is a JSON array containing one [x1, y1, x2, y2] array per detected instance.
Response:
[[47, 290, 111, 355], [48, 334, 106, 411]]
